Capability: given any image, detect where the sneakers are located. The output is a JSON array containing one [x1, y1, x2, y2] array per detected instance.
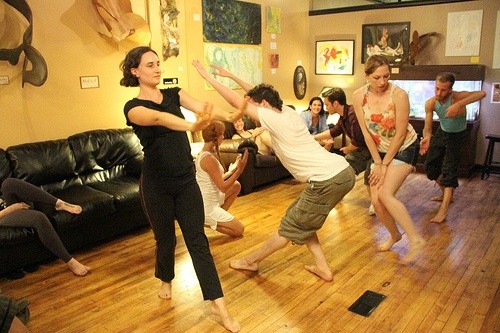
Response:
[[368, 204, 375, 216]]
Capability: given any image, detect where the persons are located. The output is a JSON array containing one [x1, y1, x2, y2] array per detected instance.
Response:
[[300, 96, 335, 151], [0, 296, 31, 333], [312, 86, 377, 217], [189, 57, 357, 282], [119, 46, 241, 332], [418, 71, 487, 224], [195, 120, 248, 236], [230, 118, 275, 156], [0, 178, 91, 276], [352, 55, 426, 265]]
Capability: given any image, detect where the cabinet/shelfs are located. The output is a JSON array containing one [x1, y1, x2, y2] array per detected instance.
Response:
[[409, 118, 481, 178]]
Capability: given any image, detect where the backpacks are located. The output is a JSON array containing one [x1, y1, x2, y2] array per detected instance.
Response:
[[0, 295, 31, 333]]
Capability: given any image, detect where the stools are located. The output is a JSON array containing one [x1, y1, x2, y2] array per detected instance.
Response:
[[482, 134, 500, 180]]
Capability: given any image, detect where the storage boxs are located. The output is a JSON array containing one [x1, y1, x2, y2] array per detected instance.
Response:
[[382, 65, 485, 122]]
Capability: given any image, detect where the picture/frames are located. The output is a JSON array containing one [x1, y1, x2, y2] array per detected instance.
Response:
[[490, 82, 500, 103], [360, 22, 411, 65], [315, 39, 355, 75]]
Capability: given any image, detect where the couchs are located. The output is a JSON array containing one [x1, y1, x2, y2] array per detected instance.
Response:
[[210, 106, 333, 194], [0, 127, 150, 282]]
